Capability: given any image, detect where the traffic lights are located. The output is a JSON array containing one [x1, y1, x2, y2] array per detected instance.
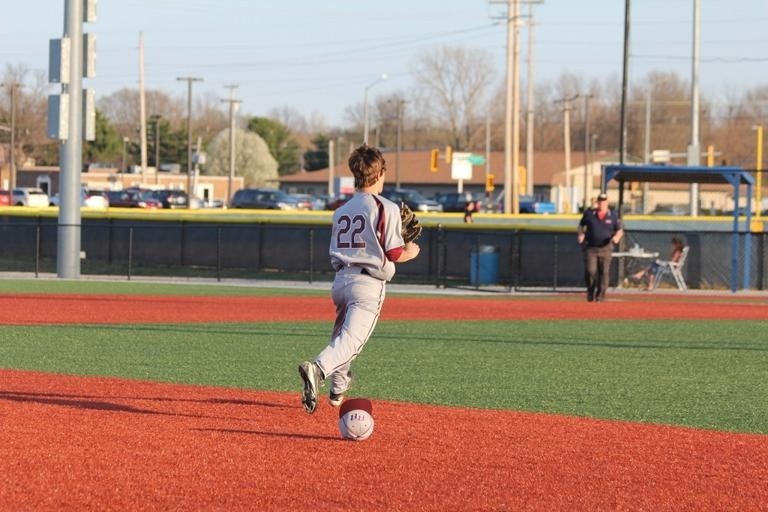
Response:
[[487, 175, 494, 191], [432, 149, 439, 172]]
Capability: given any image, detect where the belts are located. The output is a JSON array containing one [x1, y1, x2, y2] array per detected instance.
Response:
[[338, 266, 370, 275]]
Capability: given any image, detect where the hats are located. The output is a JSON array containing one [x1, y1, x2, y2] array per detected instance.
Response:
[[598, 193, 606, 201], [339, 399, 374, 441]]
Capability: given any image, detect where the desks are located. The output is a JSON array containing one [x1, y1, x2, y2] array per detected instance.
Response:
[[610, 250, 660, 291]]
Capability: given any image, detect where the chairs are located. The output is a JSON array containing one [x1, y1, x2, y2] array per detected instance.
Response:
[[652, 245, 690, 293]]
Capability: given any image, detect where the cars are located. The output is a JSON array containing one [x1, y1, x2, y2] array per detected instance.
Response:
[[327, 188, 354, 209], [648, 202, 704, 216], [437, 191, 481, 211], [10, 186, 48, 208], [0, 189, 9, 206], [288, 193, 326, 210], [109, 190, 162, 209], [212, 199, 223, 208]]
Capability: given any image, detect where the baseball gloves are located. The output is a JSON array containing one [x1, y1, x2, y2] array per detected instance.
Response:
[[396, 200, 422, 245]]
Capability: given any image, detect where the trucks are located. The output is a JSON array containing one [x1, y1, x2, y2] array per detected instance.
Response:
[[519, 195, 556, 215]]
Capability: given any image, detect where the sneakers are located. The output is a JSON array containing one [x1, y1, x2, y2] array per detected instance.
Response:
[[329, 371, 351, 407], [299, 361, 322, 414]]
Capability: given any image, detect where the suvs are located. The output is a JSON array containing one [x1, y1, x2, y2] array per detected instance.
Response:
[[387, 188, 443, 213], [49, 187, 109, 209], [231, 188, 307, 211], [152, 190, 188, 209]]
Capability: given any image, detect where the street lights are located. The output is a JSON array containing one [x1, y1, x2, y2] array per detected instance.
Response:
[[361, 74, 389, 147], [749, 124, 763, 217], [1, 80, 28, 207], [176, 75, 205, 198]]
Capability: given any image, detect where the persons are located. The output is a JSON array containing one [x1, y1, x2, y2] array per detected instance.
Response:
[[163, 194, 174, 208], [299, 143, 420, 413], [627, 238, 685, 290], [578, 195, 623, 303], [463, 202, 475, 223]]
